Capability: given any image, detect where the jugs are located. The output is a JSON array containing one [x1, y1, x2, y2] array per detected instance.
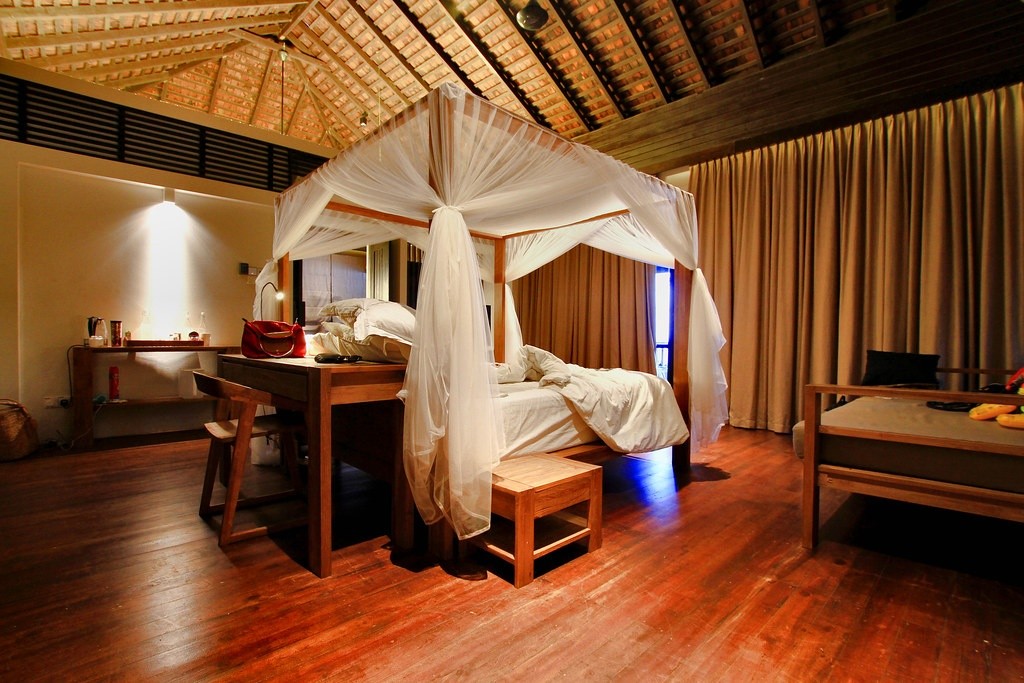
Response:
[[86, 316, 98, 336]]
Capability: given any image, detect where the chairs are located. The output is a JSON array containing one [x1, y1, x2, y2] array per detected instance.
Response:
[[193, 371, 310, 546]]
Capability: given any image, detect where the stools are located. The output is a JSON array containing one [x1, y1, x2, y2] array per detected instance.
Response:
[[458, 452, 603, 588]]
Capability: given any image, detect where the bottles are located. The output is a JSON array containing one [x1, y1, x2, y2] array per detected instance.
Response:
[[198, 312, 206, 340], [109, 366, 119, 401], [95, 318, 107, 337], [125, 331, 131, 339]]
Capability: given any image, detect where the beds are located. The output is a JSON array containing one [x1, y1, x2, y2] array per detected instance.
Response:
[[793, 368, 1024, 550], [254, 81, 728, 566]]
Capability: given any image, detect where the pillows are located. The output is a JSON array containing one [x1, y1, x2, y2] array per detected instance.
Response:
[[354, 302, 417, 346], [312, 322, 410, 364], [860, 350, 940, 389], [319, 298, 390, 328]]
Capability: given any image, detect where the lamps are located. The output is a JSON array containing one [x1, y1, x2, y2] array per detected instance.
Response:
[[277, 36, 288, 61], [359, 112, 368, 125], [162, 188, 175, 206], [516, 0, 549, 30], [260, 282, 285, 321]]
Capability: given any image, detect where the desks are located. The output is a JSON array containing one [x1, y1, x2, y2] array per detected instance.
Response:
[[218, 354, 414, 578]]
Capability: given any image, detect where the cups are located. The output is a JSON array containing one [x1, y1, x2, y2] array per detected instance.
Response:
[[111, 320, 123, 347], [201, 334, 210, 345]]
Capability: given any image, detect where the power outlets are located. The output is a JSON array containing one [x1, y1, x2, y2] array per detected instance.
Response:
[[43, 396, 71, 409]]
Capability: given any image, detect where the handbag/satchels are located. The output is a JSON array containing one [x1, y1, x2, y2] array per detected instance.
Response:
[[240, 317, 307, 359], [0, 398, 40, 463]]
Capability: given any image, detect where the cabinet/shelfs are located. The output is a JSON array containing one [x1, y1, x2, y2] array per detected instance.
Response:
[[73, 344, 241, 448]]
